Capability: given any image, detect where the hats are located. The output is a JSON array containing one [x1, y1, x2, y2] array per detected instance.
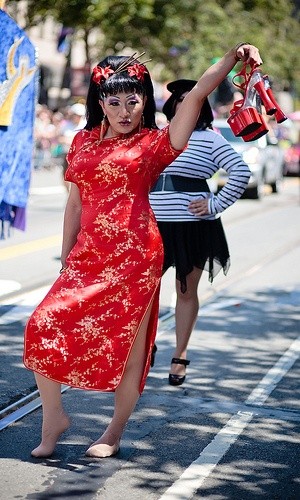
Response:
[[166, 78, 198, 94]]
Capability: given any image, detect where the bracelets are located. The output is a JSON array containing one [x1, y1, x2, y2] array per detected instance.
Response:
[[232, 41, 251, 62]]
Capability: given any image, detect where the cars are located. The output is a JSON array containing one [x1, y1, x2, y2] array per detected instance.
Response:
[[211, 117, 283, 199]]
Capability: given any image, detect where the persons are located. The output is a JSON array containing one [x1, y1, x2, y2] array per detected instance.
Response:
[[21, 38, 263, 457], [32, 100, 85, 193], [148, 80, 252, 386]]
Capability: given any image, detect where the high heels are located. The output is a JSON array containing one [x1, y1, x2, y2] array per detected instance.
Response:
[[151, 345, 157, 367], [227, 59, 277, 137], [169, 358, 190, 385], [240, 76, 287, 142]]
